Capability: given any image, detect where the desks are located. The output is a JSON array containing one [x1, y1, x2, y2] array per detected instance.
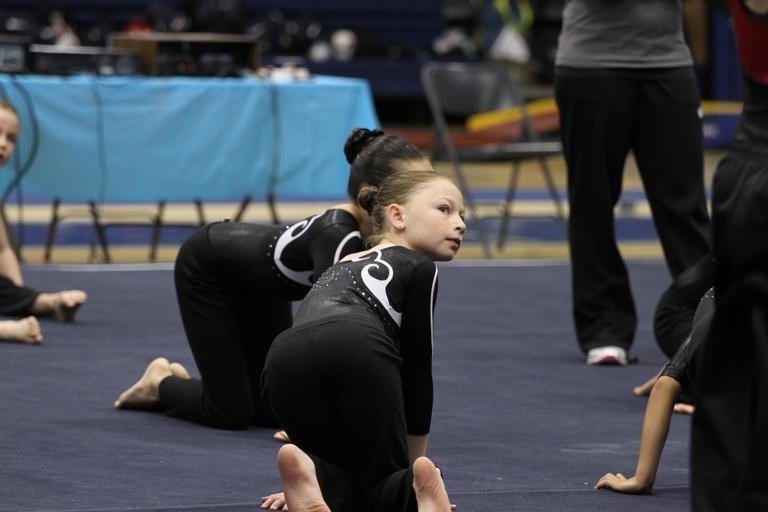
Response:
[[0, 71, 371, 264]]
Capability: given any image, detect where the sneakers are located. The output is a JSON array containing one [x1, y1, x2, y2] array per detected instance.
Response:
[[585, 343, 630, 369]]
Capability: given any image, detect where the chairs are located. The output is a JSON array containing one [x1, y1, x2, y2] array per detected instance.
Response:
[[419, 60, 566, 259]]
